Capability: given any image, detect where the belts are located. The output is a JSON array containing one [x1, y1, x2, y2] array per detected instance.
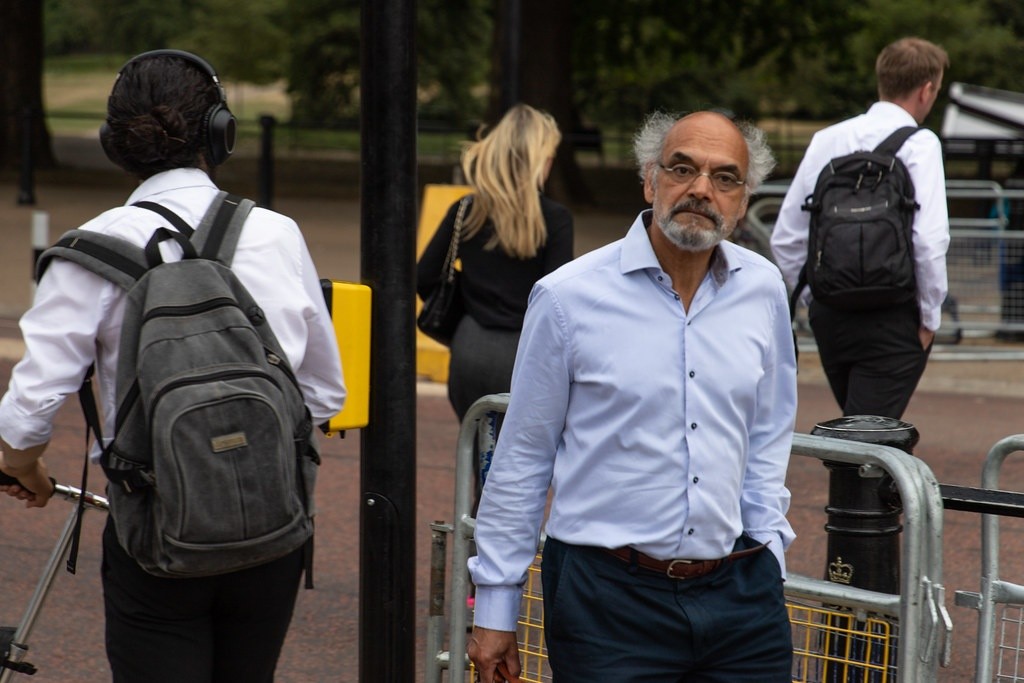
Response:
[[602, 540, 772, 579]]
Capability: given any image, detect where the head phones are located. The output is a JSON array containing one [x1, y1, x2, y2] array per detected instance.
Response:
[[99, 47, 238, 169]]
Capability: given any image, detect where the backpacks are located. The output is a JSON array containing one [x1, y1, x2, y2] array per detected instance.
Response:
[[790, 126, 925, 375], [35, 190, 322, 589]]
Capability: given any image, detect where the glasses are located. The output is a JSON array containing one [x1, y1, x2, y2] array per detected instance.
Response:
[[659, 164, 747, 192]]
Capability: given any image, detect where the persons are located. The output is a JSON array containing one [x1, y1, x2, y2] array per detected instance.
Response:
[[0, 48, 346, 683], [417, 103, 573, 629], [467, 110, 799, 683], [770, 38, 951, 419]]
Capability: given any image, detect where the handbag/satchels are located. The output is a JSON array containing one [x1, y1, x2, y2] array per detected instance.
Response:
[[417, 194, 472, 347]]
